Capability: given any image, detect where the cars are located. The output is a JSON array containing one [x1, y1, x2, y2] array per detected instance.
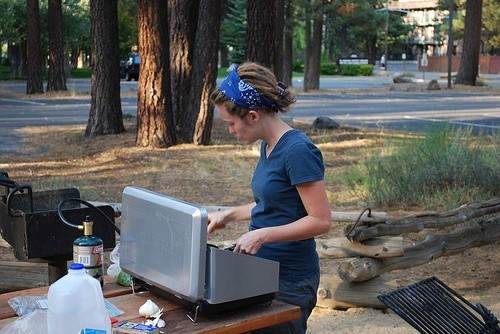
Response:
[[125, 55, 141, 81]]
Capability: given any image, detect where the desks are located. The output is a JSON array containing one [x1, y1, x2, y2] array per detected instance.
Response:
[[0, 274, 301, 334]]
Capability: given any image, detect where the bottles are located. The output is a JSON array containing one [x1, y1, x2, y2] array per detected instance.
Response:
[[46, 263, 111, 334], [73, 216, 104, 291]]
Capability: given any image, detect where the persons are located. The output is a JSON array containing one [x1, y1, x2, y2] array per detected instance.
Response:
[[207, 62, 332, 334], [381, 54, 385, 70]]
